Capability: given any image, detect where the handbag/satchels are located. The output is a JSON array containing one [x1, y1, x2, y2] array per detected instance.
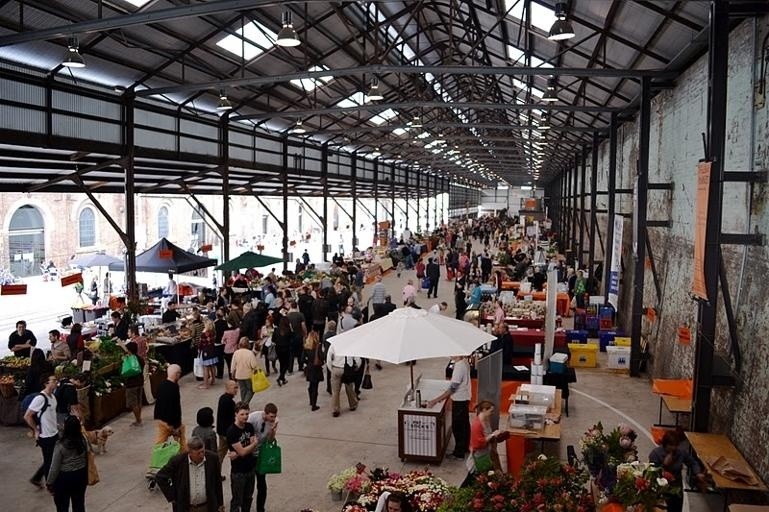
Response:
[[251, 366, 270, 393], [342, 364, 361, 382], [255, 439, 281, 474], [120, 354, 141, 378], [471, 449, 494, 474], [266, 344, 277, 362], [152, 436, 181, 468], [362, 365, 373, 389], [81, 434, 99, 486], [193, 349, 203, 378], [314, 344, 323, 367]]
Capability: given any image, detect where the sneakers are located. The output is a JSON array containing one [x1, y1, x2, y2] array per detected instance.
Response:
[[29, 480, 44, 489], [445, 454, 464, 461]]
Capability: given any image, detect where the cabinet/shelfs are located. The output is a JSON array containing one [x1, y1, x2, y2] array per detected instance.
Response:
[[397, 377, 456, 466]]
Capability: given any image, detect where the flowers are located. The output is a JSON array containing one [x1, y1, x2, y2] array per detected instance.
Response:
[[326, 420, 674, 512]]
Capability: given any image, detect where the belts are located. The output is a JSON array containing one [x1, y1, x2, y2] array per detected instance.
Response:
[[189, 502, 207, 508]]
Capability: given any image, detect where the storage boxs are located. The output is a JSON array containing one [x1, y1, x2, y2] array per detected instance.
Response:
[[505, 401, 549, 434], [560, 302, 638, 371]]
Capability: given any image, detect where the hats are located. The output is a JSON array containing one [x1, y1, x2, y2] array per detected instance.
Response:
[[125, 342, 137, 353]]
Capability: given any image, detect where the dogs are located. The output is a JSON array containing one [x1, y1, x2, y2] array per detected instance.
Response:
[[86, 425, 114, 456]]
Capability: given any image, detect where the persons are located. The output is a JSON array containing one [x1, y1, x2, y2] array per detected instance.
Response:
[[22, 416, 278, 510], [459, 399, 505, 487], [426, 354, 473, 461], [0, 211, 601, 426], [649, 427, 705, 511], [23, 373, 64, 488], [385, 490, 408, 511]]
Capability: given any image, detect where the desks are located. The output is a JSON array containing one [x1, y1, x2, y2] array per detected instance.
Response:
[[653, 377, 696, 430], [503, 384, 566, 442], [678, 430, 769, 503]]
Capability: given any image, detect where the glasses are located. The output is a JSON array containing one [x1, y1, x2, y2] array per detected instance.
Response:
[[260, 422, 265, 433]]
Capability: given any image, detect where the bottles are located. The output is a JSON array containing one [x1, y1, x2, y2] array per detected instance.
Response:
[[415, 389, 421, 407]]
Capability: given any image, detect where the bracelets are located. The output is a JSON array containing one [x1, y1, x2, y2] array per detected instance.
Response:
[[491, 432, 495, 438]]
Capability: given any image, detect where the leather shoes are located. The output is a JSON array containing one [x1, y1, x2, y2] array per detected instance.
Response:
[[333, 412, 339, 417]]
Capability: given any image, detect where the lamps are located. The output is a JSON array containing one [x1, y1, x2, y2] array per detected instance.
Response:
[[546, 0, 577, 42], [216, 87, 233, 114], [58, 32, 89, 71], [272, 8, 304, 51], [290, 75, 564, 199]]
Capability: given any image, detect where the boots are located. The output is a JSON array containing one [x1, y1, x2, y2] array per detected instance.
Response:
[[311, 393, 320, 411], [308, 390, 312, 405]]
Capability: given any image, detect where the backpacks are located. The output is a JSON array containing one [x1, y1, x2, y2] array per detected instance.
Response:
[[20, 392, 49, 427]]
